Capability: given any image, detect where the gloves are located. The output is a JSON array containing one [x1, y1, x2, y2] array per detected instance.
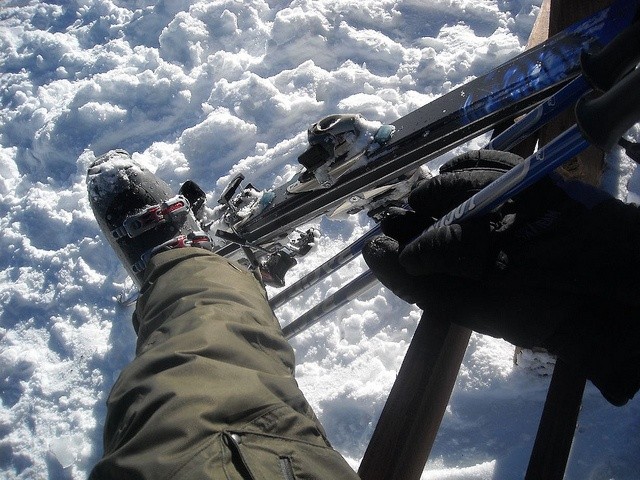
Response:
[[362, 149, 640, 406]]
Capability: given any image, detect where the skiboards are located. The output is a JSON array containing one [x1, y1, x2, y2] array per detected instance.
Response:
[[198, 0, 639, 463]]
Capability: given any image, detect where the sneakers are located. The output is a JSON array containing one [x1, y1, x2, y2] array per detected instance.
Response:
[[87, 148, 213, 285], [299, 114, 381, 182]]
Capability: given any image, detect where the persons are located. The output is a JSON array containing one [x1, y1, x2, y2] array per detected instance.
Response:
[[85, 148, 639, 480]]
